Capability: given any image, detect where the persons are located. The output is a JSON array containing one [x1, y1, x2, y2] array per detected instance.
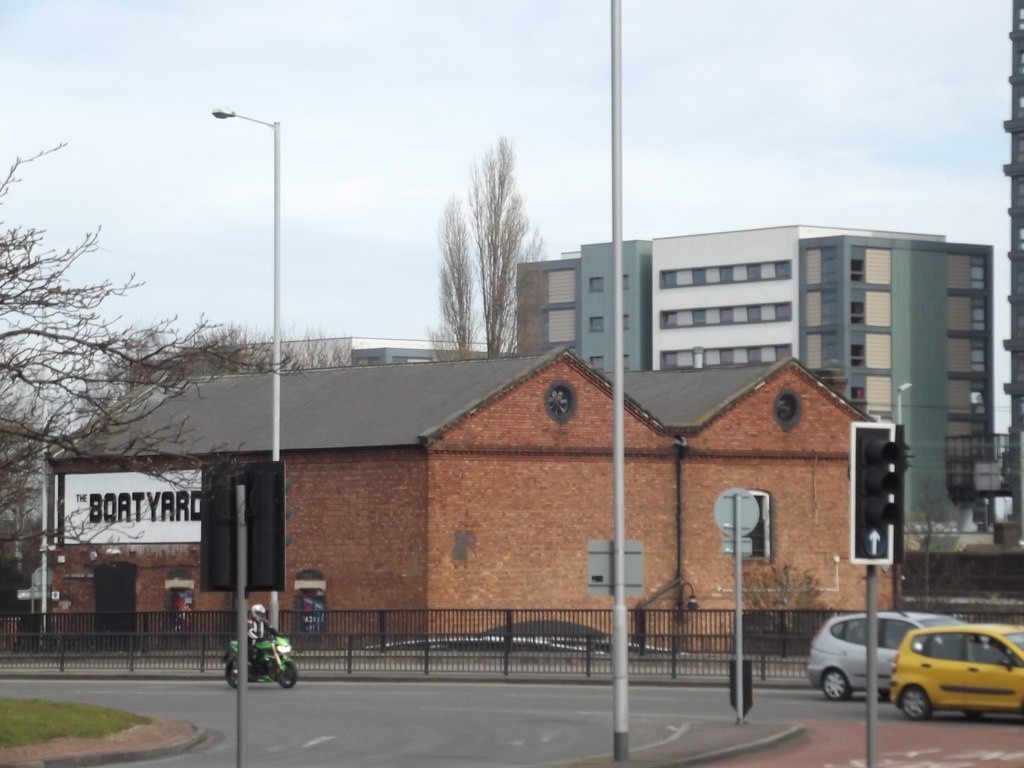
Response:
[[247, 604, 282, 680]]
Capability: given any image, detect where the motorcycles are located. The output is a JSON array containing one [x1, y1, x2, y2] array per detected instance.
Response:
[[224, 625, 299, 689]]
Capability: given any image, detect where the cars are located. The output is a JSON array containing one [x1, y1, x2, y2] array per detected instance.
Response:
[[888, 623, 1024, 718], [804, 605, 971, 702]]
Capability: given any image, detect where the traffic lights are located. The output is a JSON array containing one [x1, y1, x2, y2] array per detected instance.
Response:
[[851, 423, 902, 565]]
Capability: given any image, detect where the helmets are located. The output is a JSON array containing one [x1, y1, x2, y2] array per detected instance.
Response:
[[250, 604, 266, 622]]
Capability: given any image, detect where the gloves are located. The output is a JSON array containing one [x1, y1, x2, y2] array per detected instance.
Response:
[[256, 638, 264, 642]]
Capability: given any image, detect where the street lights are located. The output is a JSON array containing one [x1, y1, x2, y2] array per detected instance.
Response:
[[895, 382, 914, 425], [212, 109, 280, 635]]
